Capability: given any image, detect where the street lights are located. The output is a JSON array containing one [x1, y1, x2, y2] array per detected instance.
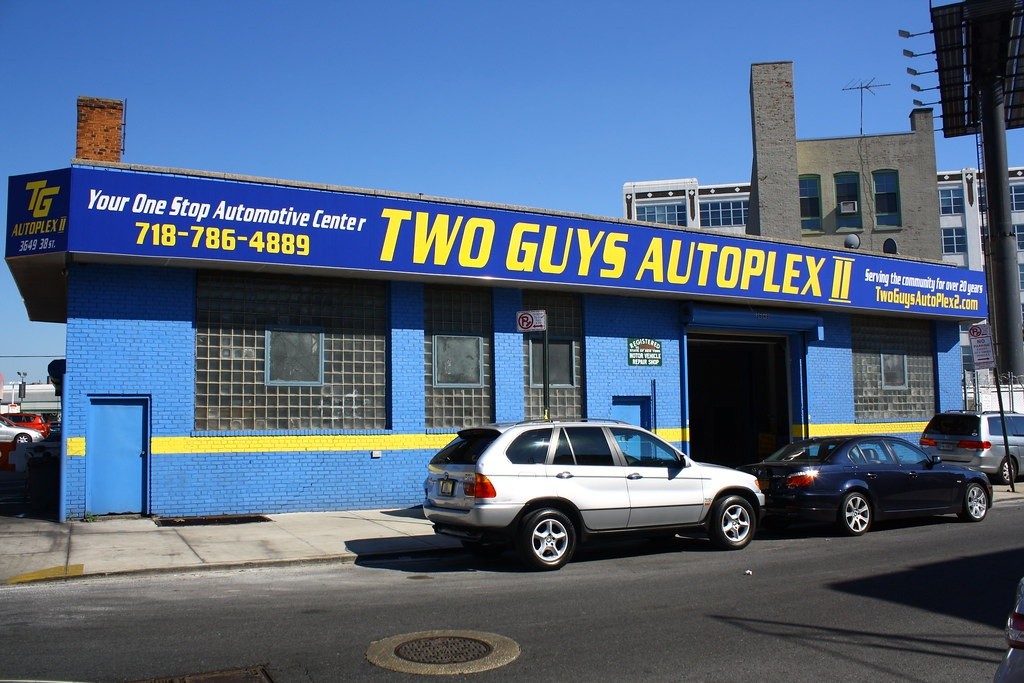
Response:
[[17, 371, 27, 402], [9, 382, 20, 404]]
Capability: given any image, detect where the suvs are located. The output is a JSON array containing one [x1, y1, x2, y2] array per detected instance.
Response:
[[918, 409, 1024, 489], [422, 415, 766, 570], [2, 413, 50, 439]]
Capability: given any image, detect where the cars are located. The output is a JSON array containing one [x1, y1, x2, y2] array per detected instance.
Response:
[[735, 434, 994, 535], [0, 418, 44, 443]]
